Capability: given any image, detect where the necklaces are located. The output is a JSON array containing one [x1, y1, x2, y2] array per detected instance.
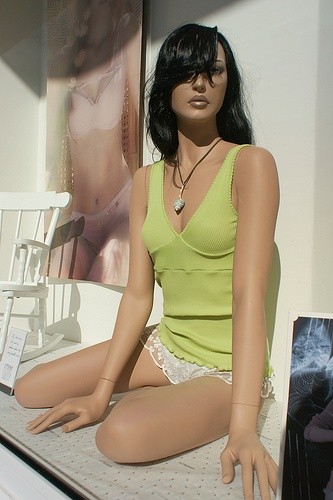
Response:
[[173, 137, 223, 212]]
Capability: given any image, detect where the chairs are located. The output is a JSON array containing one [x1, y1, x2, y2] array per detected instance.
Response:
[[0, 189, 73, 364]]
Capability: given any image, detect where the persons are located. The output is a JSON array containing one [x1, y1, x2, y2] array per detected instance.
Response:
[[44, 1, 146, 293], [15, 22, 277, 498]]
